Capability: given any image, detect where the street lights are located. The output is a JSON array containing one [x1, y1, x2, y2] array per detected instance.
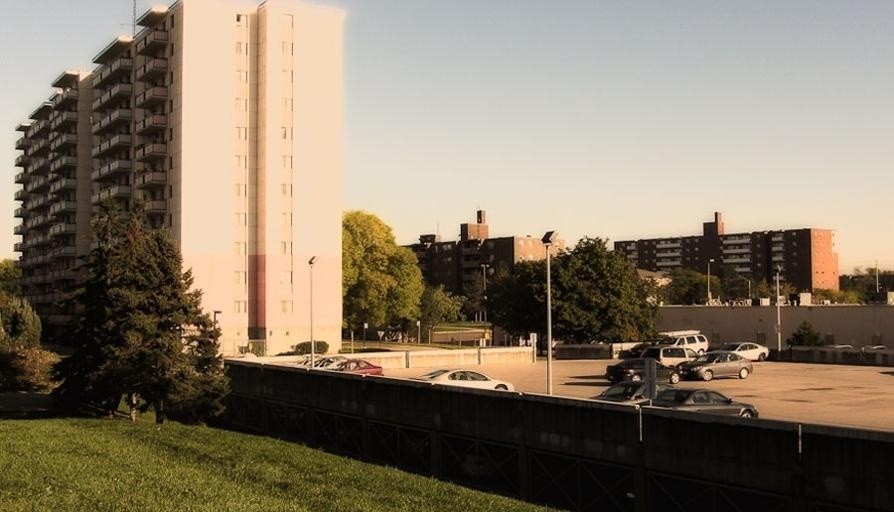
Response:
[[481, 263, 490, 322], [707, 258, 715, 302], [541, 231, 558, 395], [775, 265, 783, 352], [307, 255, 318, 369]]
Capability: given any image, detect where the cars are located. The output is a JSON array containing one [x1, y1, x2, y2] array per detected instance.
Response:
[[292, 353, 384, 376], [408, 368, 515, 392], [598, 333, 769, 418]]
